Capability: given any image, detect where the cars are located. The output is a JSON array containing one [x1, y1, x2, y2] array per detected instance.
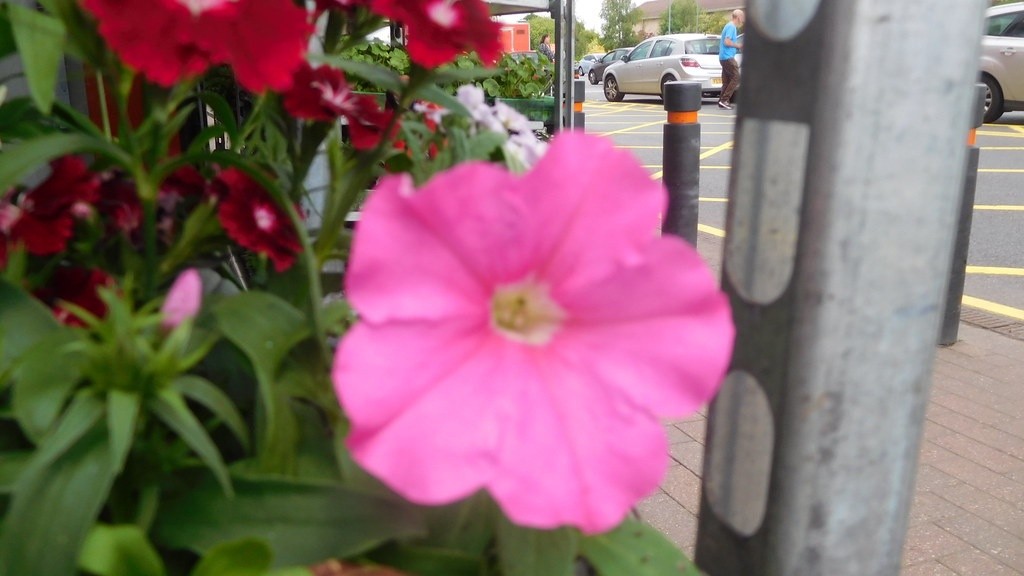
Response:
[[587, 47, 636, 85], [601, 33, 743, 103], [505, 49, 545, 79], [976, 1, 1024, 124], [577, 53, 608, 76]]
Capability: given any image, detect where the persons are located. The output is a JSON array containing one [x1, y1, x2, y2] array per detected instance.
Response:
[[717, 9, 744, 110], [538, 34, 555, 96]]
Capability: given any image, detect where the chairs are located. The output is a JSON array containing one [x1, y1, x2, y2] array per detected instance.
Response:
[[660, 47, 672, 56], [707, 45, 719, 53]]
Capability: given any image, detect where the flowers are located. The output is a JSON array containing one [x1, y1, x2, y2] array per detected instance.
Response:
[[0, 0, 736, 576]]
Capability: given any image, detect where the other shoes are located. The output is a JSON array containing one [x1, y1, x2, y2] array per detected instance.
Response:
[[719, 101, 733, 110]]
